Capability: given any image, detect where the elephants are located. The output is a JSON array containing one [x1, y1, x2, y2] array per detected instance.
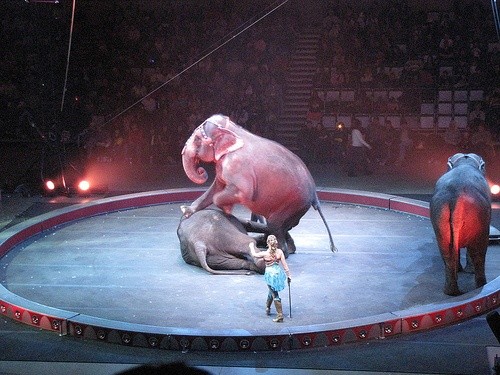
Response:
[[430, 152, 492, 296], [180, 114, 338, 259], [177, 207, 272, 276]]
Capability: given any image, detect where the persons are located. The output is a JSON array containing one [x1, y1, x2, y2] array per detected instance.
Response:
[[249, 234, 292, 323], [0, 0, 499, 174]]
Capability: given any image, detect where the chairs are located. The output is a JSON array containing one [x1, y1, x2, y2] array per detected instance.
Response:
[[67, 0, 500, 176]]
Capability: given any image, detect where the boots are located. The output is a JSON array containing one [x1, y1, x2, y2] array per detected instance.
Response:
[[266, 296, 273, 314], [272, 298, 283, 322]]
